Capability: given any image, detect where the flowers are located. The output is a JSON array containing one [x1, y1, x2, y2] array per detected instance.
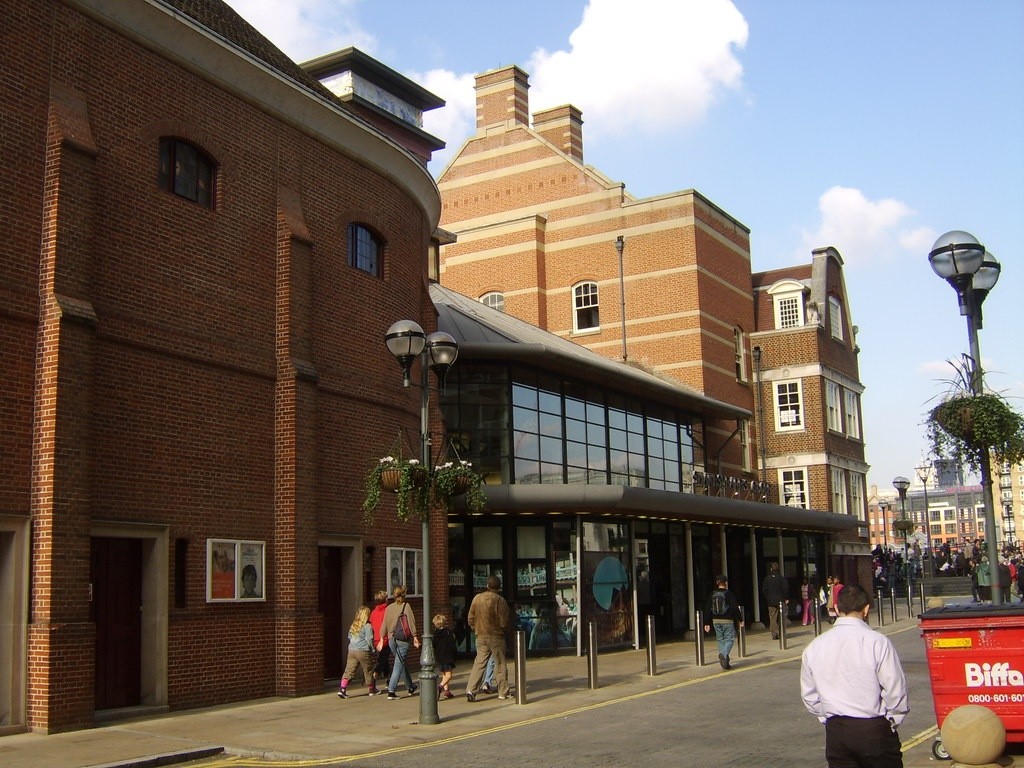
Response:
[[434, 461, 489, 518], [355, 432, 431, 531]]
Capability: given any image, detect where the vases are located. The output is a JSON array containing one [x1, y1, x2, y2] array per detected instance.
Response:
[[379, 468, 423, 494], [438, 474, 470, 498]]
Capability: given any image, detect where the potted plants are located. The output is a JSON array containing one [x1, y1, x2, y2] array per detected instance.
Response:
[[918, 355, 1024, 479], [892, 520, 915, 537]]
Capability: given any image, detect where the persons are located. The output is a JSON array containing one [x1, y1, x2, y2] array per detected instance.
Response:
[[465, 575, 515, 701], [367, 590, 393, 689], [530, 593, 577, 647], [761, 561, 790, 640], [336, 606, 382, 698], [240, 565, 260, 598], [515, 602, 535, 650], [704, 574, 745, 670], [430, 614, 457, 700], [379, 584, 422, 700], [801, 577, 815, 626], [871, 537, 1024, 606], [819, 574, 844, 624], [799, 582, 910, 768]]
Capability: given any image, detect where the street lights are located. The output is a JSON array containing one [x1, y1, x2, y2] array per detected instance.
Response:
[[928, 230, 1001, 606], [1001, 497, 1013, 541], [915, 462, 936, 577], [384, 320, 459, 725], [878, 498, 888, 553], [893, 477, 914, 578]]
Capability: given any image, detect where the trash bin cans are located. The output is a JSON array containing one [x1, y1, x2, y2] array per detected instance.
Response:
[[923, 555, 934, 577], [916, 603, 1024, 759]]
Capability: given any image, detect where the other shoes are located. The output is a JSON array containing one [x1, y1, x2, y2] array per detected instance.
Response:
[[725, 665, 731, 670], [408, 684, 419, 696], [466, 691, 476, 702], [371, 675, 377, 688], [483, 683, 493, 693], [498, 691, 515, 700], [436, 684, 442, 700], [369, 687, 381, 696], [444, 692, 454, 698], [387, 692, 402, 700], [385, 679, 400, 690], [337, 687, 349, 699], [773, 635, 780, 640], [718, 653, 727, 669]]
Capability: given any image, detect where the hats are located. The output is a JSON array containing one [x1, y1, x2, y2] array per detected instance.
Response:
[[714, 575, 727, 582]]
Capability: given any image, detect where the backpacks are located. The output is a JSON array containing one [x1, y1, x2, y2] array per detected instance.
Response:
[[393, 602, 413, 642]]
